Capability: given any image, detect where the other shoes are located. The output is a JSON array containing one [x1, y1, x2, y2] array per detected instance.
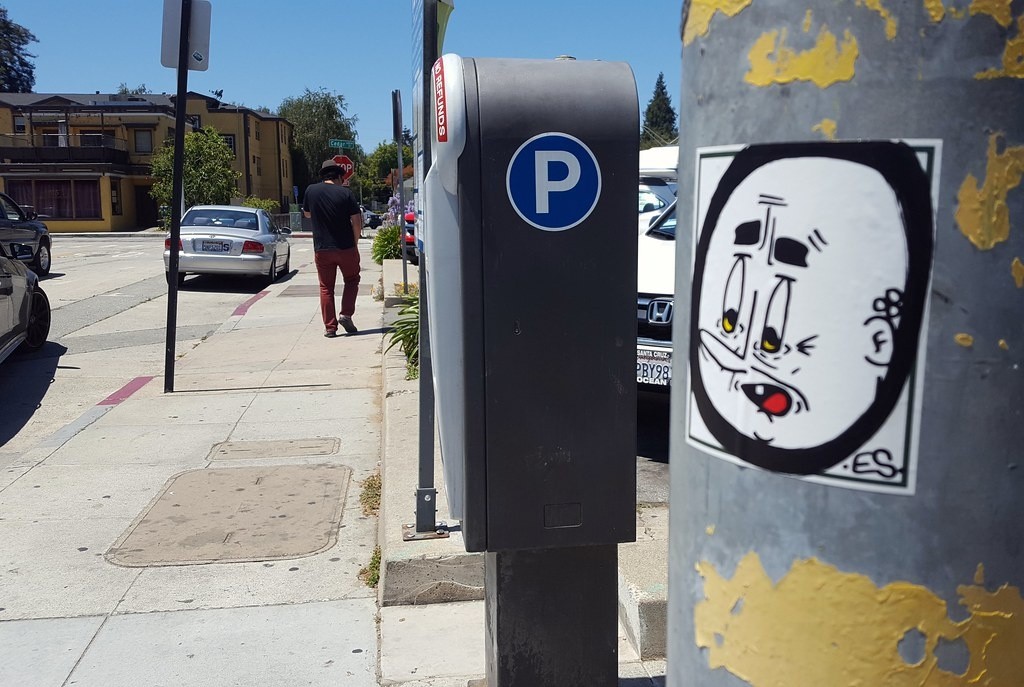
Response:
[[339, 315, 357, 333], [324, 331, 336, 338]]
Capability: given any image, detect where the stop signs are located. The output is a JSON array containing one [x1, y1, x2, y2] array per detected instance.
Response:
[[329, 154, 355, 183]]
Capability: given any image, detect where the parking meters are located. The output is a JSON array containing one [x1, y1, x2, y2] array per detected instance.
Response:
[[415, 50, 641, 685]]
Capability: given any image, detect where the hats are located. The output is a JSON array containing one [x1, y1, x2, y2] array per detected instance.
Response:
[[319, 160, 346, 177]]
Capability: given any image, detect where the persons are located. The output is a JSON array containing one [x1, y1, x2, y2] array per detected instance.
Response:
[[303, 159, 361, 338]]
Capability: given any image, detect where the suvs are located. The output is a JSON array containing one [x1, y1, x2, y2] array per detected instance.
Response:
[[633, 140, 681, 435]]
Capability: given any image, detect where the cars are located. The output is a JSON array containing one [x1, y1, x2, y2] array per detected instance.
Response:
[[0, 243, 53, 365], [360, 207, 383, 230], [162, 204, 292, 286], [399, 212, 418, 267], [0, 195, 54, 279]]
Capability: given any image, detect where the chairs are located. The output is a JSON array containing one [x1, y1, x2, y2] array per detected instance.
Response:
[[234, 221, 252, 229], [194, 217, 212, 226]]
